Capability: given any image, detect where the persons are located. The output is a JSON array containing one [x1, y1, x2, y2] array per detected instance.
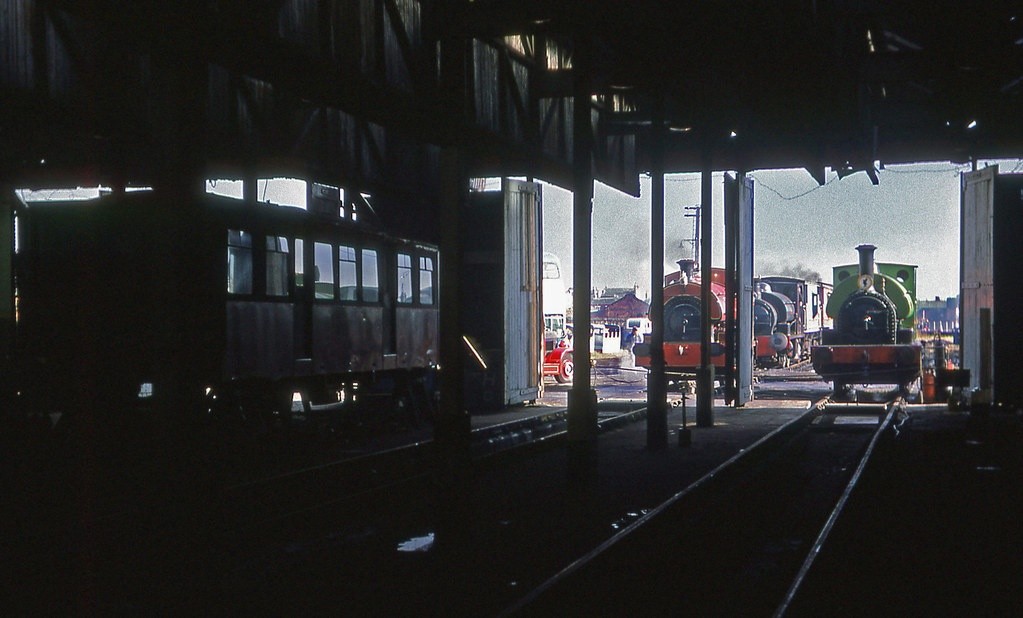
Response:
[[624, 326, 641, 352]]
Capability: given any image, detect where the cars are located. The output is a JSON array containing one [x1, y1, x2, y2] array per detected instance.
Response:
[[544, 331, 574, 384]]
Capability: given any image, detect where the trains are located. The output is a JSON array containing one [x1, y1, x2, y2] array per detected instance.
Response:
[[632, 260, 835, 392], [16, 173, 440, 454], [811, 244, 924, 399]]
